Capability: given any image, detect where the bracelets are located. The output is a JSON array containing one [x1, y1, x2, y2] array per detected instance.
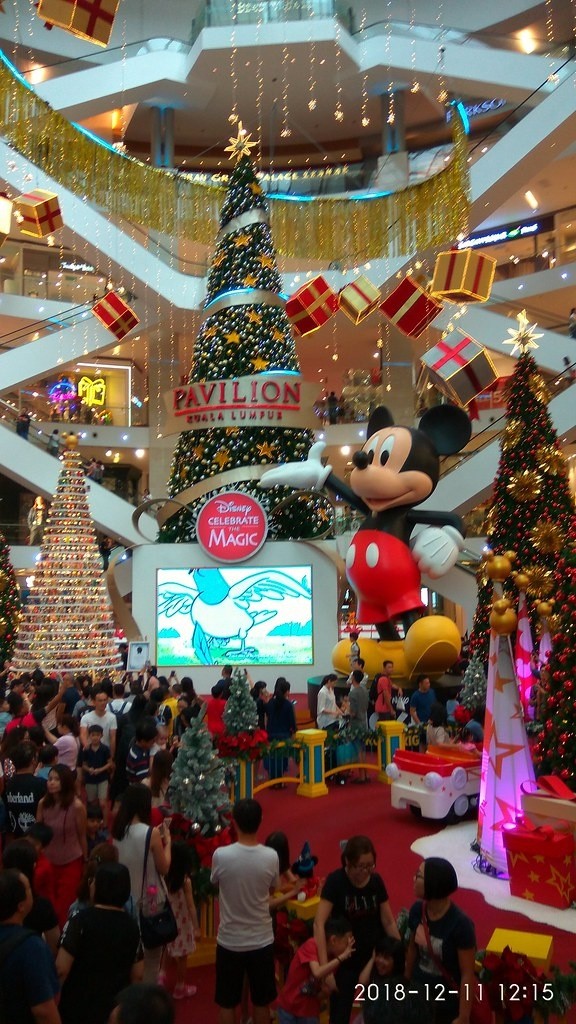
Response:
[[337, 956, 342, 962]]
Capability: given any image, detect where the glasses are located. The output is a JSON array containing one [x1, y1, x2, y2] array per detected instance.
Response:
[[87, 878, 96, 887], [415, 870, 424, 881], [350, 860, 376, 873]]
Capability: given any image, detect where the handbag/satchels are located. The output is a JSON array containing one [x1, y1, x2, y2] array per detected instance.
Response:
[[465, 973, 496, 1024], [140, 900, 179, 951]]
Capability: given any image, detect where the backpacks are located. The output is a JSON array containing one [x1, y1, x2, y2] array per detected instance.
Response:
[[369, 672, 388, 702]]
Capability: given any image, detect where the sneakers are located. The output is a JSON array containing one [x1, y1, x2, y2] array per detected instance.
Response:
[[158, 975, 170, 992], [174, 985, 196, 999]]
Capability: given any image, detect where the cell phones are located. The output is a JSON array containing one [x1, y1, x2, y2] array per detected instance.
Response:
[[160, 817, 172, 838]]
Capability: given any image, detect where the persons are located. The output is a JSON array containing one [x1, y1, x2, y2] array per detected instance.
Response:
[[90, 404, 106, 424], [258, 836, 400, 1024], [141, 489, 153, 514], [315, 657, 402, 785], [0, 658, 204, 840], [27, 496, 47, 546], [404, 857, 479, 1024], [0, 740, 200, 1024], [83, 456, 106, 486], [208, 663, 296, 790], [561, 355, 574, 386], [408, 674, 484, 754], [45, 429, 60, 457], [208, 796, 280, 1023], [13, 406, 31, 442], [357, 935, 411, 1024], [342, 504, 357, 529], [347, 631, 360, 670], [569, 308, 576, 338], [328, 391, 346, 425], [100, 535, 111, 571]]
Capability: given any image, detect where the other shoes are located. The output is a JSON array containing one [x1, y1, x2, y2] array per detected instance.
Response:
[[269, 783, 286, 790], [349, 777, 367, 784]]
